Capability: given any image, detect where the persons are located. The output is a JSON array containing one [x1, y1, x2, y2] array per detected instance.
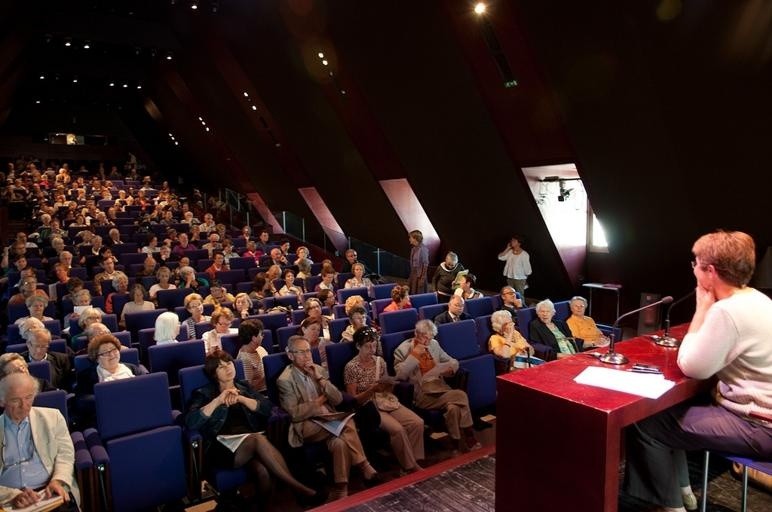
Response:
[[527, 297, 580, 362], [566, 295, 612, 351], [0, 150, 485, 512], [495, 232, 534, 309], [623, 228, 772, 512], [497, 284, 525, 311], [487, 309, 536, 377]]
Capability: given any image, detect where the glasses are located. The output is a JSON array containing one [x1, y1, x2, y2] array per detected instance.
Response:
[[217, 321, 232, 328], [362, 327, 377, 335], [96, 346, 120, 360], [289, 349, 315, 356], [501, 292, 517, 295]]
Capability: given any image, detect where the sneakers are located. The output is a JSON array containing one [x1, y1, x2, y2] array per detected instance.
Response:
[[299, 442, 481, 509]]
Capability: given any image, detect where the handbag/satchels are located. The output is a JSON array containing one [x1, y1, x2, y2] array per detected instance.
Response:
[[372, 392, 401, 412]]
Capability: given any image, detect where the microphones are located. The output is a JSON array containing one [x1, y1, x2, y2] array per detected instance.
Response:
[[655, 288, 697, 347], [598, 295, 674, 365]]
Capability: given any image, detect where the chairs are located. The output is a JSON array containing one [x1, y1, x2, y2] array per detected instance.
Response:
[[702, 446, 772, 512]]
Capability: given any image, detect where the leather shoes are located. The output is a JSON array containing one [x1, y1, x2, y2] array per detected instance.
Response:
[[682, 493, 697, 511]]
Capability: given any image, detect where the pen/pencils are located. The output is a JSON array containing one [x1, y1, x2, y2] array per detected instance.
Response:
[[626, 365, 663, 374]]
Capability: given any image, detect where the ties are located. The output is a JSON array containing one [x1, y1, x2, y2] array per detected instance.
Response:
[[304, 375, 330, 415]]
